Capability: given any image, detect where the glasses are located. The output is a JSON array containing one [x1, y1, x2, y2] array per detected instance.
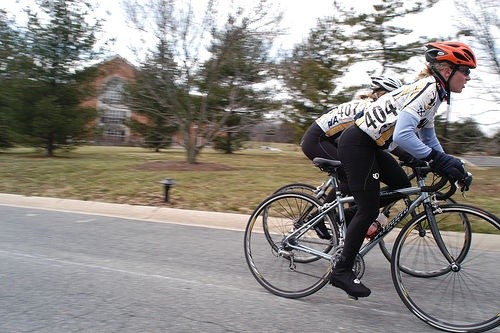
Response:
[[450, 63, 471, 76]]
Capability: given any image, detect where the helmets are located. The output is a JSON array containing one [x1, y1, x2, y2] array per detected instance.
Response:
[[423, 41, 477, 70], [371, 75, 403, 93]]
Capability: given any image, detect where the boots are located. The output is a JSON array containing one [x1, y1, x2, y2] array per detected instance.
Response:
[[331, 252, 372, 298], [333, 205, 358, 228]]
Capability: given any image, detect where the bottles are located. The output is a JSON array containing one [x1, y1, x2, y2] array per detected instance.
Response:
[[366, 210, 390, 242]]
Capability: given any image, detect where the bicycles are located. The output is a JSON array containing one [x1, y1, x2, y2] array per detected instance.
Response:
[[243, 156, 500, 333], [262, 161, 473, 277]]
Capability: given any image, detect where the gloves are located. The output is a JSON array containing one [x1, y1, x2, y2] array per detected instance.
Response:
[[456, 171, 473, 193], [432, 151, 467, 181], [398, 150, 428, 178]]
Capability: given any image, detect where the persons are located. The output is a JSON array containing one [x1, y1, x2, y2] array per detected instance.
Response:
[[328, 42, 477, 298], [300, 79, 400, 239]]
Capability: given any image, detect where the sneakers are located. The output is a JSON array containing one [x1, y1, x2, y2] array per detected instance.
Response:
[[308, 210, 332, 240]]
